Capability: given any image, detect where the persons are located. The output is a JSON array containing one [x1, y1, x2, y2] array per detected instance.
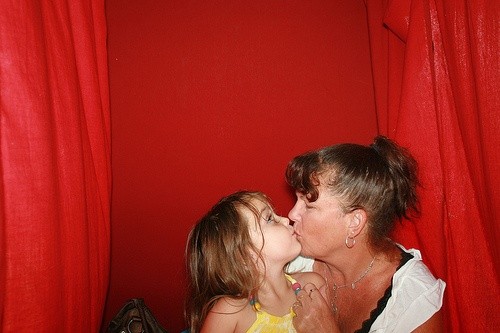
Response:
[[285, 136, 446, 333], [186, 192, 327, 333]]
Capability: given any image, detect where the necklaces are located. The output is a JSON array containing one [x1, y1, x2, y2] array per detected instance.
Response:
[[330, 258, 375, 311]]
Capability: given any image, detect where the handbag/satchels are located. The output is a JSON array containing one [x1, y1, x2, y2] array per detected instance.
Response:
[[108, 298, 166, 333]]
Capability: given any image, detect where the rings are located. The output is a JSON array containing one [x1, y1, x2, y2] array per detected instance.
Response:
[[292, 287, 319, 311]]
